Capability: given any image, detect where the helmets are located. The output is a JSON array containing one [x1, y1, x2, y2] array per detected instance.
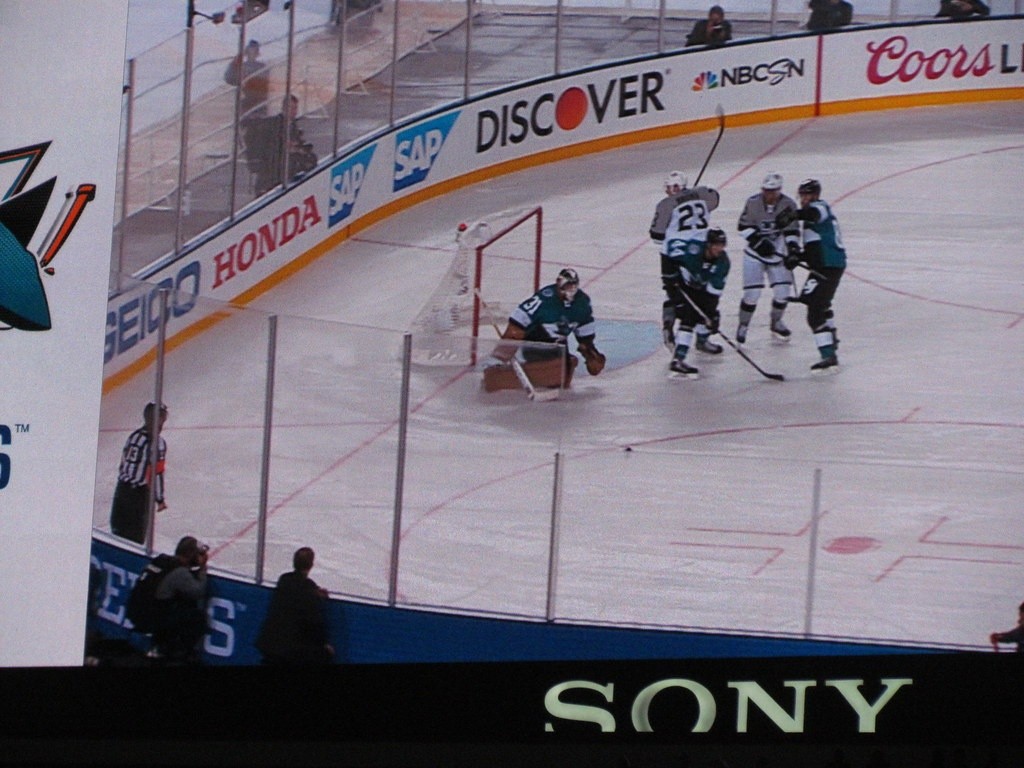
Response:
[[798, 179, 822, 194], [665, 170, 688, 187], [761, 173, 784, 189], [558, 269, 580, 288], [708, 227, 727, 247]]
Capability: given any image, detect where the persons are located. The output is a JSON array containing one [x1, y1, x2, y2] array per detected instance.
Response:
[[258, 548, 333, 664], [128, 537, 211, 659], [666, 227, 731, 379], [936, 0, 989, 16], [736, 174, 800, 349], [808, 0, 852, 30], [224, 40, 298, 196], [686, 6, 731, 47], [990, 603, 1024, 652], [651, 171, 720, 352], [482, 268, 605, 390], [111, 403, 168, 544], [776, 179, 847, 376]]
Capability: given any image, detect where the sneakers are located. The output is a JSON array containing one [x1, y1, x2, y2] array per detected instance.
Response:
[[667, 360, 700, 381], [735, 322, 748, 348], [812, 355, 840, 376], [694, 334, 726, 357], [770, 319, 793, 341]]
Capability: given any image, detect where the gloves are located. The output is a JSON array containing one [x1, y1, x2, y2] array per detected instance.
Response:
[[783, 254, 803, 269], [748, 237, 775, 259], [664, 284, 685, 305], [774, 206, 792, 228], [705, 309, 720, 330]]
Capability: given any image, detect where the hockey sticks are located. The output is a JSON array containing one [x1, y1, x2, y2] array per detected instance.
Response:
[[773, 250, 852, 286], [473, 286, 560, 402], [692, 102, 726, 187], [676, 283, 787, 383]]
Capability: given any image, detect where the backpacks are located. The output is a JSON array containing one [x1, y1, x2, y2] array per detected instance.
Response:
[[127, 557, 176, 633]]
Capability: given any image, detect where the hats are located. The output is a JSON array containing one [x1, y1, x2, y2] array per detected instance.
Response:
[[176, 536, 209, 559]]
